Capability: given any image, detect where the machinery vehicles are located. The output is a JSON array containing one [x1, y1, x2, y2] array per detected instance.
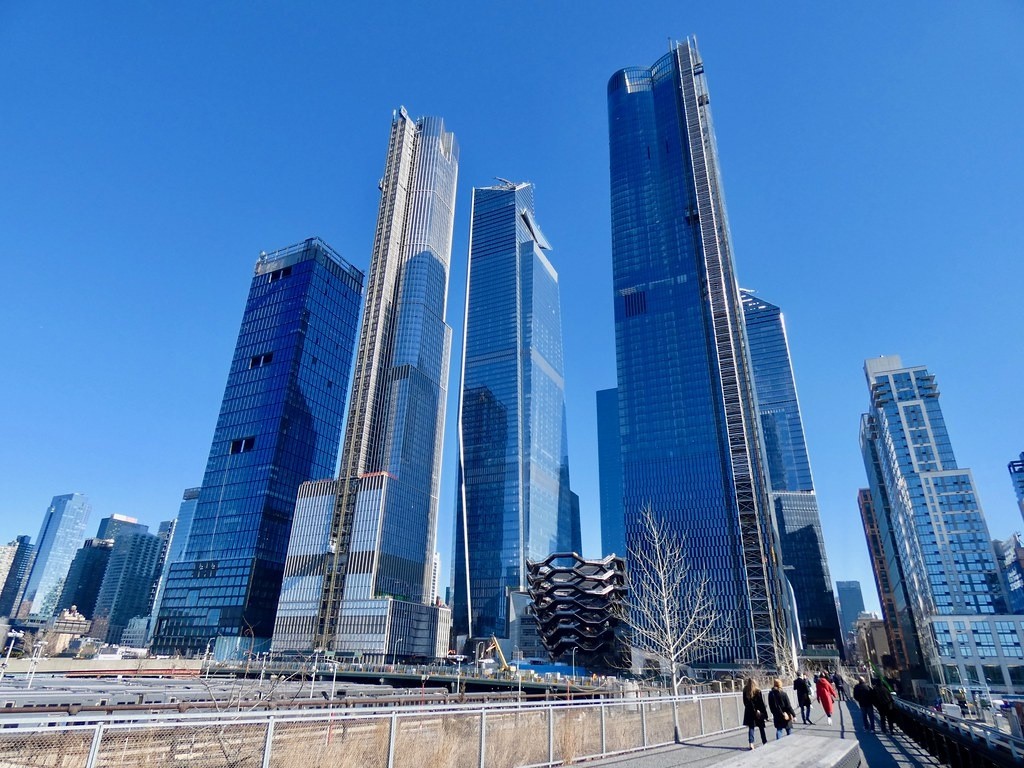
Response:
[[484, 632, 517, 681]]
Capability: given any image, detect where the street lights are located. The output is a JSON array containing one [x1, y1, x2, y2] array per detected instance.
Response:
[[572, 647, 578, 680], [27, 641, 49, 689], [0, 632, 24, 680], [25, 644, 41, 680], [476, 641, 484, 673], [392, 638, 403, 665]]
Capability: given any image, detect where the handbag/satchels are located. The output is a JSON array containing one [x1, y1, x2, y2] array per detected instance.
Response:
[[756, 710, 761, 719], [784, 712, 793, 720], [831, 695, 835, 703]]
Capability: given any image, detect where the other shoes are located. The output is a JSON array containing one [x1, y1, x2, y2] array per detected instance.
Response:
[[749, 743, 754, 749]]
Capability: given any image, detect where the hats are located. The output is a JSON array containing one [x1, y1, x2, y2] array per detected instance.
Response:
[[859, 676, 865, 682]]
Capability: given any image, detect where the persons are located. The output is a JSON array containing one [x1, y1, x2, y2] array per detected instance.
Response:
[[768, 679, 797, 740], [793, 670, 896, 735], [743, 678, 769, 750]]
